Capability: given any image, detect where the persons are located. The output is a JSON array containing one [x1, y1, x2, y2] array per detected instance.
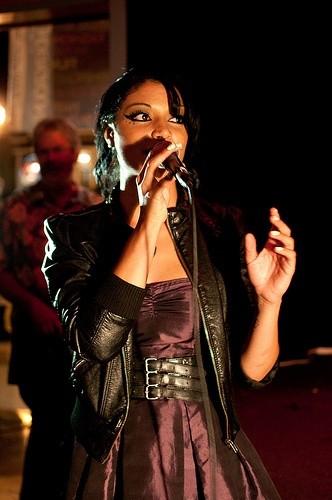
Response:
[[0, 118, 136, 499], [41, 73, 304, 500]]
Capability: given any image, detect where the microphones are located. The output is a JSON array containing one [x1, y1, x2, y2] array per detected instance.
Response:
[[152, 140, 193, 189]]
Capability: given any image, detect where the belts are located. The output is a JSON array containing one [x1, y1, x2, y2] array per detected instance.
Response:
[[131, 356, 203, 401]]
